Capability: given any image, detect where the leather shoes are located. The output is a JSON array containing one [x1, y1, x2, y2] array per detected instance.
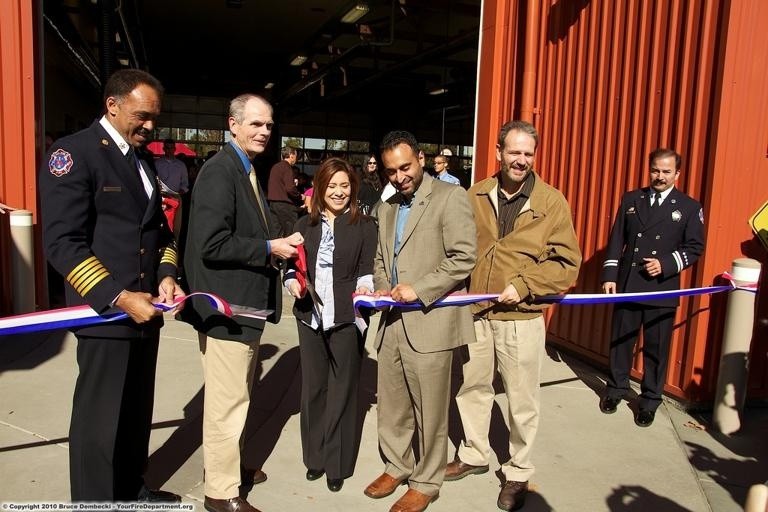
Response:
[[497, 481, 528, 511], [306, 469, 325, 481], [144, 488, 182, 504], [326, 478, 344, 492], [600, 395, 621, 414], [635, 409, 655, 427], [204, 496, 261, 512], [363, 473, 407, 499], [389, 488, 439, 512], [443, 455, 489, 481], [241, 468, 267, 485]]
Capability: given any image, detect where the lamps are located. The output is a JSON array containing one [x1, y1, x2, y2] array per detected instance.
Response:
[[284, 42, 311, 68], [334, 2, 369, 25]]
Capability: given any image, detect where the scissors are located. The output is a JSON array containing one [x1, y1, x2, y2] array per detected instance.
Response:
[[294, 245, 323, 320]]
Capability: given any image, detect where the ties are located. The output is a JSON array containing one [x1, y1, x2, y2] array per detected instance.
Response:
[[249, 164, 267, 221], [651, 192, 661, 208], [127, 145, 144, 186]]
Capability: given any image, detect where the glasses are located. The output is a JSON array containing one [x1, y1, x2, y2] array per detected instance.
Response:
[[433, 161, 444, 165], [368, 162, 376, 164]]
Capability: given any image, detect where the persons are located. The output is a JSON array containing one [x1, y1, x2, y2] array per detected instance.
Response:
[[175, 95, 304, 512], [363, 132, 477, 511], [138, 138, 220, 245], [355, 154, 387, 216], [251, 147, 315, 237], [430, 153, 459, 186], [39, 68, 186, 504], [601, 150, 703, 426], [445, 120, 584, 512], [282, 157, 380, 493]]
